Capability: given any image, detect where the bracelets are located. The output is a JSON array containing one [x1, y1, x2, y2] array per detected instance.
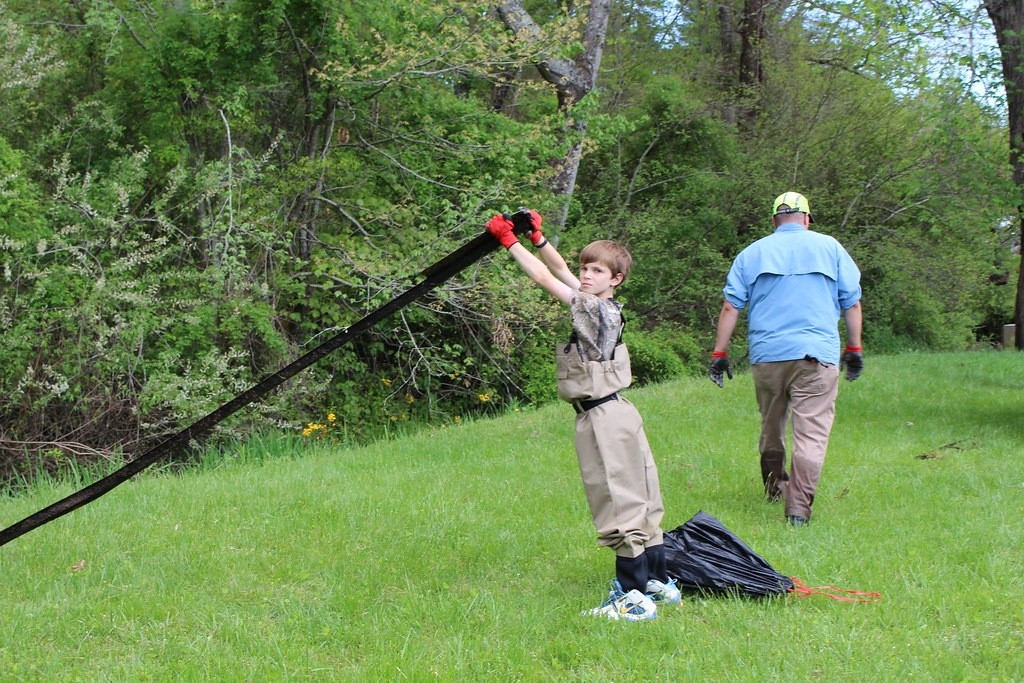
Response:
[[535, 238, 547, 248]]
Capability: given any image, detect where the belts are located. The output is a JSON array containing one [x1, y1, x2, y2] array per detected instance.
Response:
[[572, 391, 617, 414]]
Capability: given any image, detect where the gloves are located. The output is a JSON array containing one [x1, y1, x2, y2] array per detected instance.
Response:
[[485, 213, 520, 251], [841, 345, 864, 382], [709, 352, 733, 389], [526, 207, 543, 244]]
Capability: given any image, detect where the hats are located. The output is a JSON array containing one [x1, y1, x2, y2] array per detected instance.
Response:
[[772, 192, 815, 223]]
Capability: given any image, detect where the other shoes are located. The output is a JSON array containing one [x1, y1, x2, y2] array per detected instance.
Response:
[[786, 515, 810, 527]]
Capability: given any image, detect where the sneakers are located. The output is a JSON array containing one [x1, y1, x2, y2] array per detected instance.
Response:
[[578, 577, 658, 623], [644, 576, 683, 607]]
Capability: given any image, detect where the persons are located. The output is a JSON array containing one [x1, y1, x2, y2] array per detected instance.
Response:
[[488, 208, 680, 620], [709, 191, 862, 527]]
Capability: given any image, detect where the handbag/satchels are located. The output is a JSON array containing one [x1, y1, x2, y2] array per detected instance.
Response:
[[662, 508, 796, 601]]
